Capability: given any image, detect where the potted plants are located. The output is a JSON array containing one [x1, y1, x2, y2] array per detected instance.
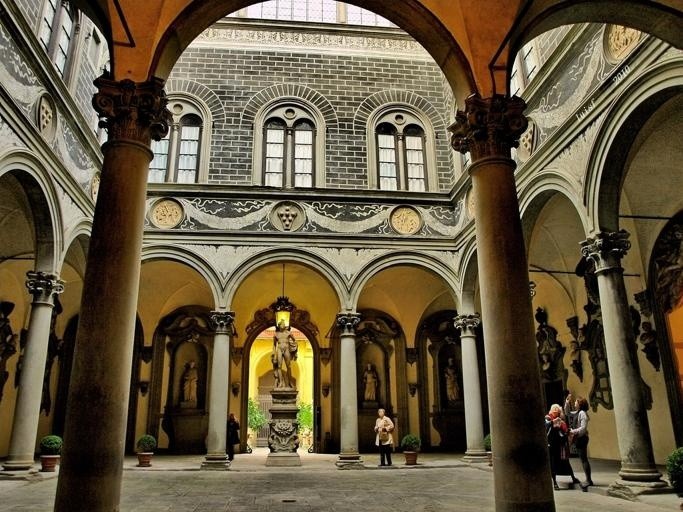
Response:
[[480, 433, 492, 467], [37, 434, 62, 470], [134, 434, 158, 467], [400, 432, 421, 464]]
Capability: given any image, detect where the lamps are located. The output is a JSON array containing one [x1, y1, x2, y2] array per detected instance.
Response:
[[272, 263, 295, 331]]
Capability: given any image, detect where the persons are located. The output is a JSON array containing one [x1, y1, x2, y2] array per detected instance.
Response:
[[180, 359, 200, 402], [544, 404, 581, 489], [564, 394, 593, 488], [568, 341, 582, 382], [226, 413, 240, 461], [640, 321, 660, 371], [444, 356, 461, 401], [374, 408, 394, 466], [363, 363, 379, 401], [270, 321, 298, 389]]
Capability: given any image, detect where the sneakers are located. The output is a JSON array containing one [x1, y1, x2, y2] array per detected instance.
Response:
[[573, 478, 593, 489], [554, 484, 560, 490]]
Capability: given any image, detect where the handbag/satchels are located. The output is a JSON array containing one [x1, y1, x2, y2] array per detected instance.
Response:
[[379, 432, 389, 441]]
